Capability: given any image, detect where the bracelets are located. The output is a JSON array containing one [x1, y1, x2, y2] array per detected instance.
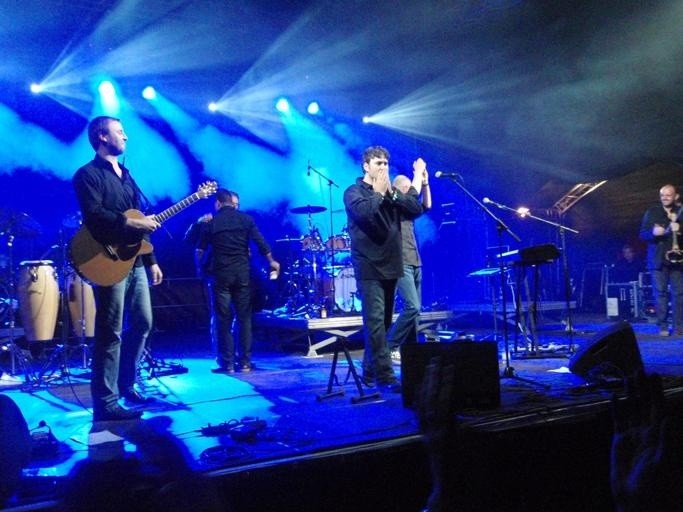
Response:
[[423, 184, 429, 186]]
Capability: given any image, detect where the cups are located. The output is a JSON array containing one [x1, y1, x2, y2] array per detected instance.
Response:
[[501, 352, 510, 364], [269, 270, 277, 281]]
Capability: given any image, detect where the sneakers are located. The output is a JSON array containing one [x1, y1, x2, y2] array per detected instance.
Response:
[[390, 350, 402, 365], [660, 324, 683, 339], [215, 357, 254, 374], [91, 398, 145, 422], [119, 386, 146, 403], [359, 363, 404, 394]]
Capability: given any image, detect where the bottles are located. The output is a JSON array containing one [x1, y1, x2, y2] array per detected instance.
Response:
[[320, 305, 326, 318]]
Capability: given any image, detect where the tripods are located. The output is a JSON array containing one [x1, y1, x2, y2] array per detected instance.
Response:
[[451, 178, 551, 391], [479, 274, 525, 346], [0, 271, 152, 387], [275, 168, 346, 318]]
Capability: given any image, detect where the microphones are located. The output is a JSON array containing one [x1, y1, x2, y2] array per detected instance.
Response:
[[483, 197, 503, 209], [435, 171, 457, 178], [307, 160, 312, 176]]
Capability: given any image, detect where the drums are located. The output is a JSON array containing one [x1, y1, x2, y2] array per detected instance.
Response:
[[303, 234, 321, 250], [325, 265, 363, 314], [65, 273, 97, 337], [326, 235, 349, 255], [20, 260, 60, 342]]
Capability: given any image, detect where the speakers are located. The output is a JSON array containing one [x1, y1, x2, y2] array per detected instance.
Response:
[[569, 321, 643, 387], [400, 339, 499, 414]]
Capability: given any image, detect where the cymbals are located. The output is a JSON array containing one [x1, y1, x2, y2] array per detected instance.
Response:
[[290, 205, 327, 214], [276, 238, 302, 242]]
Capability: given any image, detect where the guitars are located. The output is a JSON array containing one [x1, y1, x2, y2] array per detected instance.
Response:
[[70, 182, 221, 287]]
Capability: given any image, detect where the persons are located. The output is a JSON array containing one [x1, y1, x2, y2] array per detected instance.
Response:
[[196, 189, 280, 374], [612, 244, 642, 312], [71, 116, 164, 420], [386, 169, 433, 362], [184, 191, 251, 362], [344, 145, 423, 391], [638, 185, 683, 336], [417, 354, 683, 512]]
